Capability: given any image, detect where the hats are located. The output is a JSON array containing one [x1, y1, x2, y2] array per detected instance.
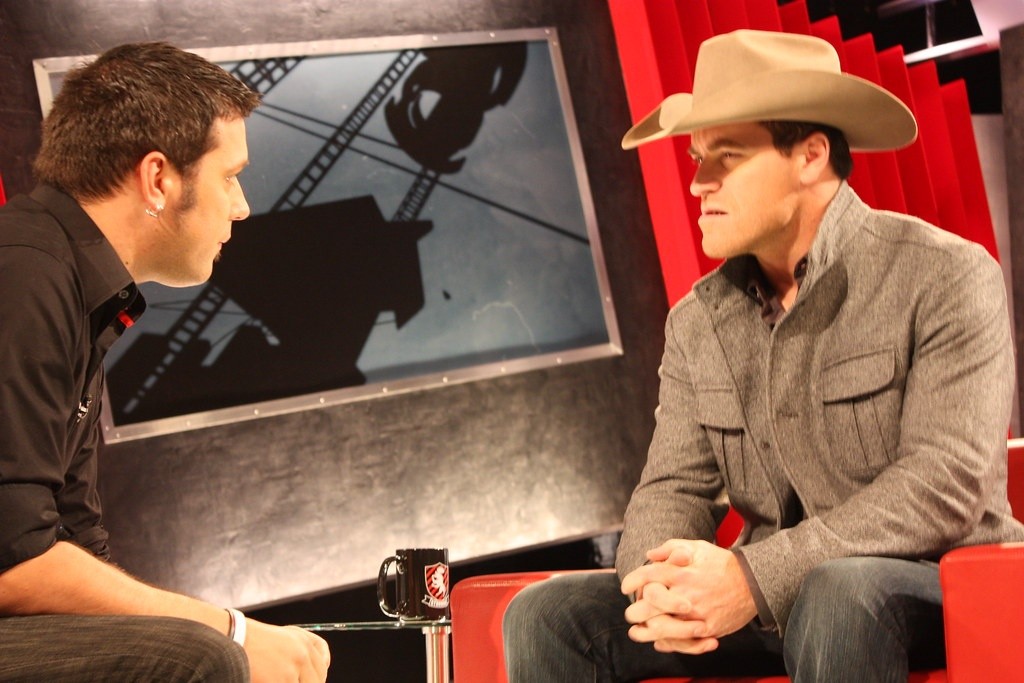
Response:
[[620, 27, 918, 152]]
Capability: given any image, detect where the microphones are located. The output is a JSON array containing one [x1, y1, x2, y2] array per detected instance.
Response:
[[76, 394, 93, 423]]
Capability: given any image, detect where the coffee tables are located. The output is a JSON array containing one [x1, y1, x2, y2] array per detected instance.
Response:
[[273, 618, 452, 683]]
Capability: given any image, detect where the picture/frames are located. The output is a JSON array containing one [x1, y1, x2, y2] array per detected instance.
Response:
[[34, 27, 623, 444]]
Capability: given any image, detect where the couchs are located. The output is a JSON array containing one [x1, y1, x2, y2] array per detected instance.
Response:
[[450, 445, 1024, 683]]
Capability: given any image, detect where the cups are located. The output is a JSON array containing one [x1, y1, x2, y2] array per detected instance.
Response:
[[377, 549, 450, 623]]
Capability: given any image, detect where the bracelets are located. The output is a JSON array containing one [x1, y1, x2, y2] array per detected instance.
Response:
[[225, 607, 246, 647]]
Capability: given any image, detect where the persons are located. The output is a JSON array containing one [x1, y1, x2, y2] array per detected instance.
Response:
[[502, 28, 1024, 683], [1, 41, 332, 683]]
